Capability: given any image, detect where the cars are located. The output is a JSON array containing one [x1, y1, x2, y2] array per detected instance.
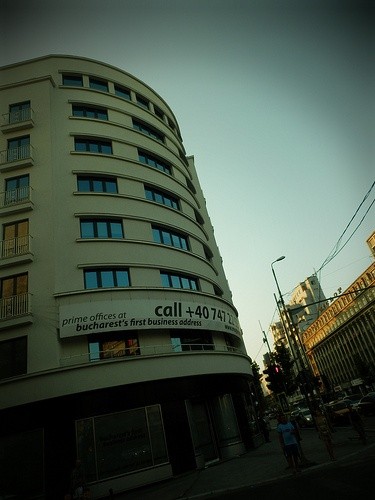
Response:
[[264, 391, 365, 428], [355, 392, 375, 417]]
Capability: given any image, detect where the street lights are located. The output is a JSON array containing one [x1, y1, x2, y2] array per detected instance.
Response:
[[269, 255, 317, 400]]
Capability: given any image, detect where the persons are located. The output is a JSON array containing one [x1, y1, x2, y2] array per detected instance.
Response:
[[347, 404, 369, 446], [277, 415, 307, 476], [259, 416, 272, 443], [312, 409, 337, 462], [71, 458, 85, 500]]
